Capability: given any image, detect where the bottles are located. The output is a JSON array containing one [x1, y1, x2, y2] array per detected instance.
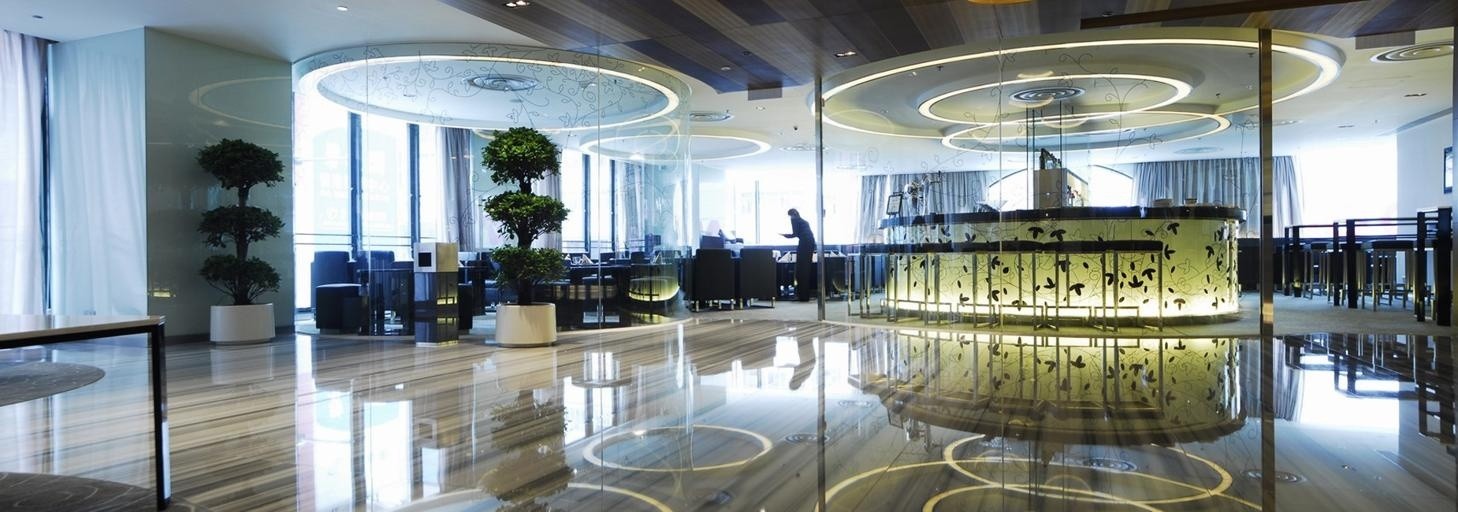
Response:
[[649, 254, 656, 265]]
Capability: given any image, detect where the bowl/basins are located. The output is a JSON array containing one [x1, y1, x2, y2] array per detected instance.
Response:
[[1153, 199, 1174, 206], [1185, 199, 1197, 204]]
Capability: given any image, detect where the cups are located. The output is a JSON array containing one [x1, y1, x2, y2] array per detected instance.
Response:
[[573, 256, 584, 265]]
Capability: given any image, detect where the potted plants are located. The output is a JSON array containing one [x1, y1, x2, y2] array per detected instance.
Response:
[[209, 345, 275, 433], [196, 138, 285, 343], [483, 346, 571, 512], [482, 127, 567, 347]]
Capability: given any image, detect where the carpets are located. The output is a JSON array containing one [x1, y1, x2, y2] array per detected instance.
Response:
[[0, 360, 105, 406], [0, 471, 213, 512]]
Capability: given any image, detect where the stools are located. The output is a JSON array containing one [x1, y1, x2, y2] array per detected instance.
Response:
[[1301, 330, 1457, 398], [1283, 235, 1455, 316]]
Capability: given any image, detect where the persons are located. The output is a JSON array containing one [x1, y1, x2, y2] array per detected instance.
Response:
[[780, 208, 815, 301]]
